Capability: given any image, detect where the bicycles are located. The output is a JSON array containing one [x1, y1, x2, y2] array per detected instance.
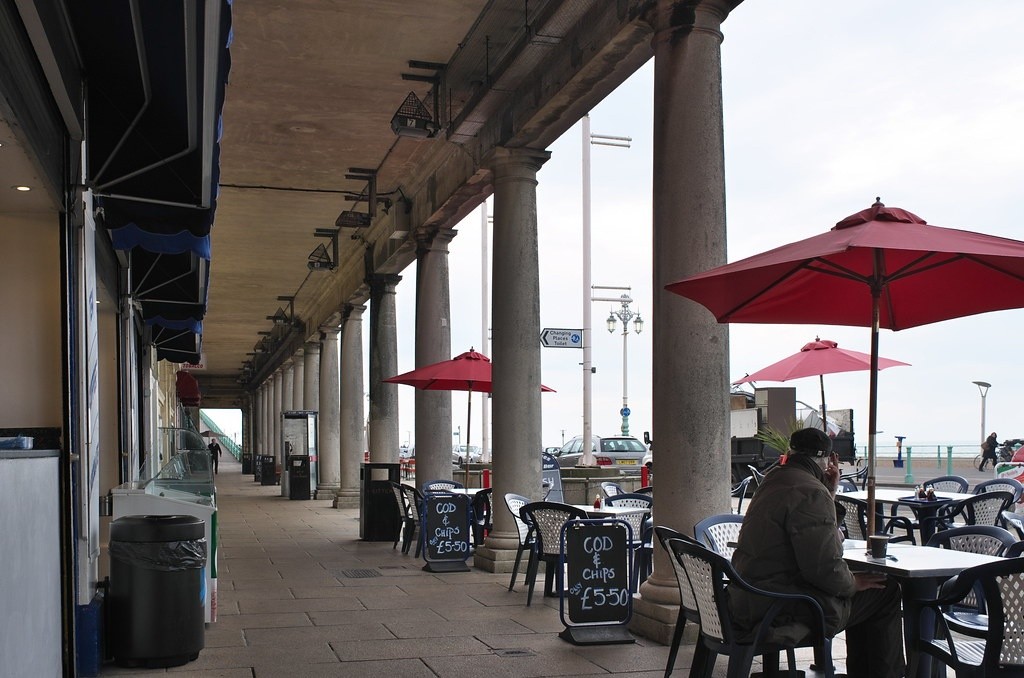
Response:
[[974, 452, 994, 471]]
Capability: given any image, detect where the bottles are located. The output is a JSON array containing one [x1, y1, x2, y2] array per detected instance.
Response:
[[915, 484, 934, 500], [594, 494, 605, 511]]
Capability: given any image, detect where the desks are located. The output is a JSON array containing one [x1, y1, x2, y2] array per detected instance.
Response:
[[840, 489, 976, 546], [570, 503, 650, 519], [842, 539, 1011, 678], [432, 488, 486, 497]]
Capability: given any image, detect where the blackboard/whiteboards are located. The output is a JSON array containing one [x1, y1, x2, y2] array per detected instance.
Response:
[[426, 497, 469, 559], [566, 525, 627, 622]]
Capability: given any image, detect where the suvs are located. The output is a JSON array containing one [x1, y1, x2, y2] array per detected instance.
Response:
[[542, 432, 654, 474]]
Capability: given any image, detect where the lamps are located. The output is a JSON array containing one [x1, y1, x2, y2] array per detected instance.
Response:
[[307, 243, 334, 271], [336, 210, 371, 228], [243, 306, 287, 370], [390, 91, 432, 140]]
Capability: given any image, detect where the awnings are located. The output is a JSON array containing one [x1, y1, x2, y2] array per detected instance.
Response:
[[177, 371, 199, 402], [143, 320, 203, 364], [112, 227, 210, 321], [66, 0, 234, 226], [182, 392, 201, 407]]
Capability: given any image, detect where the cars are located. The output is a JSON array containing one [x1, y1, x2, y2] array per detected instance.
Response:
[[730, 434, 783, 497], [398, 445, 414, 458], [452, 444, 479, 468], [469, 446, 492, 463]]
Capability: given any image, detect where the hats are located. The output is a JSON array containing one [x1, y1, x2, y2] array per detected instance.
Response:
[[790, 427, 833, 457]]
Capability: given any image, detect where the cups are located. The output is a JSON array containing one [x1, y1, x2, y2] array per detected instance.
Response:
[[869, 536, 890, 560]]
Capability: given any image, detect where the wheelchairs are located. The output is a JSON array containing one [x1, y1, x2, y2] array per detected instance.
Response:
[[995, 446, 1008, 463]]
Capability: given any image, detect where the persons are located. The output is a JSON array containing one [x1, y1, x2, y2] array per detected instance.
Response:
[[725, 428, 907, 678], [208, 439, 222, 474], [1001, 440, 1015, 466], [978, 432, 999, 471]]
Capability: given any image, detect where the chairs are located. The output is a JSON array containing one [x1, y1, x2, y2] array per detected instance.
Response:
[[504, 481, 654, 607], [655, 464, 1024, 678], [389, 479, 493, 558]]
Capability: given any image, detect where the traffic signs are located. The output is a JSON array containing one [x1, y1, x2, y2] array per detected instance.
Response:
[[539, 328, 582, 349]]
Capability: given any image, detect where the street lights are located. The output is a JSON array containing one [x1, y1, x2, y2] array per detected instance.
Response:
[[971, 381, 992, 454], [605, 294, 643, 436]]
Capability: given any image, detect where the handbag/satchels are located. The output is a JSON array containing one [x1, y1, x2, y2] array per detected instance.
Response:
[[980, 441, 992, 452]]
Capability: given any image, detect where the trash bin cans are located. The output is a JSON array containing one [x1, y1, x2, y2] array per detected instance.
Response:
[[261, 455, 276, 486], [110, 514, 206, 669], [359, 462, 402, 541], [289, 455, 311, 501], [242, 453, 252, 474]]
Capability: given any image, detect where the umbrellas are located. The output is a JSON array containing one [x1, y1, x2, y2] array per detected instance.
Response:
[[200, 430, 228, 444], [732, 334, 913, 432], [664, 198, 1024, 548], [382, 346, 557, 487]]
